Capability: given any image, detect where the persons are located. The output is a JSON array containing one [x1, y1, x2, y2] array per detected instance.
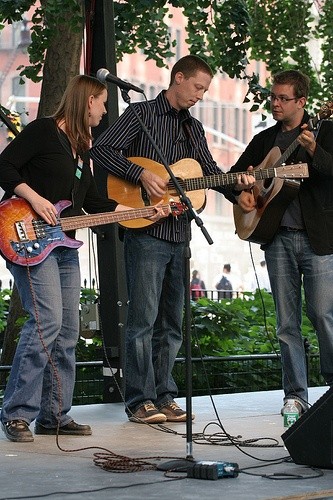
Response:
[[89, 55, 256, 424], [216, 264, 233, 301], [190, 269, 207, 301], [255, 261, 270, 292], [225, 70, 333, 416], [0, 75, 172, 442]]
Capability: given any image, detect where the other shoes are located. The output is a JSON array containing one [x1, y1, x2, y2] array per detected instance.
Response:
[[281, 399, 302, 416]]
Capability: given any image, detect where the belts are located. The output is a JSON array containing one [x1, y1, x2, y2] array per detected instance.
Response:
[[279, 226, 299, 232]]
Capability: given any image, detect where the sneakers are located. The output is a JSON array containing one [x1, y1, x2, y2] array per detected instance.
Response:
[[158, 401, 195, 422], [0, 418, 34, 442], [34, 420, 92, 434], [128, 404, 167, 424]]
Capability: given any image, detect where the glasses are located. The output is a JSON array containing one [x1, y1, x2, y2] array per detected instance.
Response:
[[265, 95, 296, 105]]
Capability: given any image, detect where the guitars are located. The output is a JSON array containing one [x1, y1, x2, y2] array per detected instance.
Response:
[[233, 99, 333, 245], [0, 197, 187, 267], [107, 156, 309, 231]]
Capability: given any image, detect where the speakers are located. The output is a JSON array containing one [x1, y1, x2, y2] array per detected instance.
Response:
[[281, 382, 333, 470]]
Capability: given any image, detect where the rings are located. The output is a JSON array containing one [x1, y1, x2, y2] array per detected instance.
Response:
[[50, 211, 53, 214]]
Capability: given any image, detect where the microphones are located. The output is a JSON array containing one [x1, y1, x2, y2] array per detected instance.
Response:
[[96, 68, 144, 94]]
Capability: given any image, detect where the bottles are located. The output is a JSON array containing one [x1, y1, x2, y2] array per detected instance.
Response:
[[283, 399, 300, 451]]
